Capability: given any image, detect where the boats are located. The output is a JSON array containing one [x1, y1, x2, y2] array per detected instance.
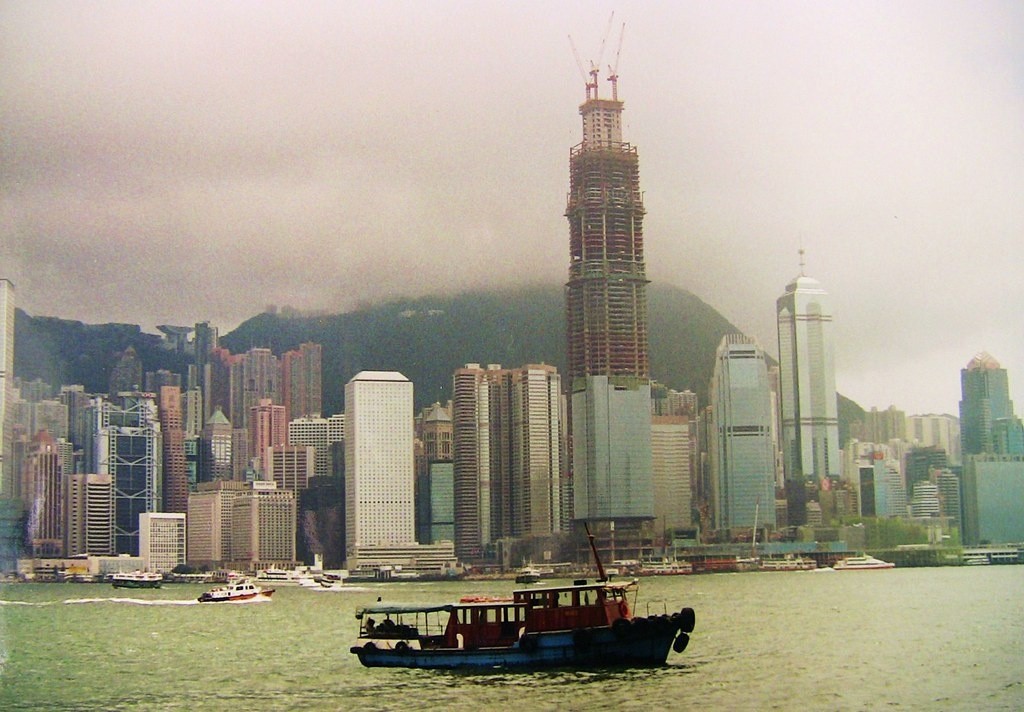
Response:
[[516, 566, 541, 583], [252, 568, 344, 590], [112, 570, 164, 588], [197, 578, 277, 602], [204, 570, 228, 583], [351, 520, 695, 669], [568, 496, 817, 581], [833, 550, 896, 570]]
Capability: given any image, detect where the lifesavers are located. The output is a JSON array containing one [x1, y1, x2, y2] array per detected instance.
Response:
[[680, 608, 695, 632], [394, 641, 408, 649], [613, 617, 633, 642], [673, 633, 689, 652]]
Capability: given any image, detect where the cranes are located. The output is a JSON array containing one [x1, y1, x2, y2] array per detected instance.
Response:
[[589, 10, 615, 100], [607, 21, 626, 101], [567, 35, 597, 102]]
